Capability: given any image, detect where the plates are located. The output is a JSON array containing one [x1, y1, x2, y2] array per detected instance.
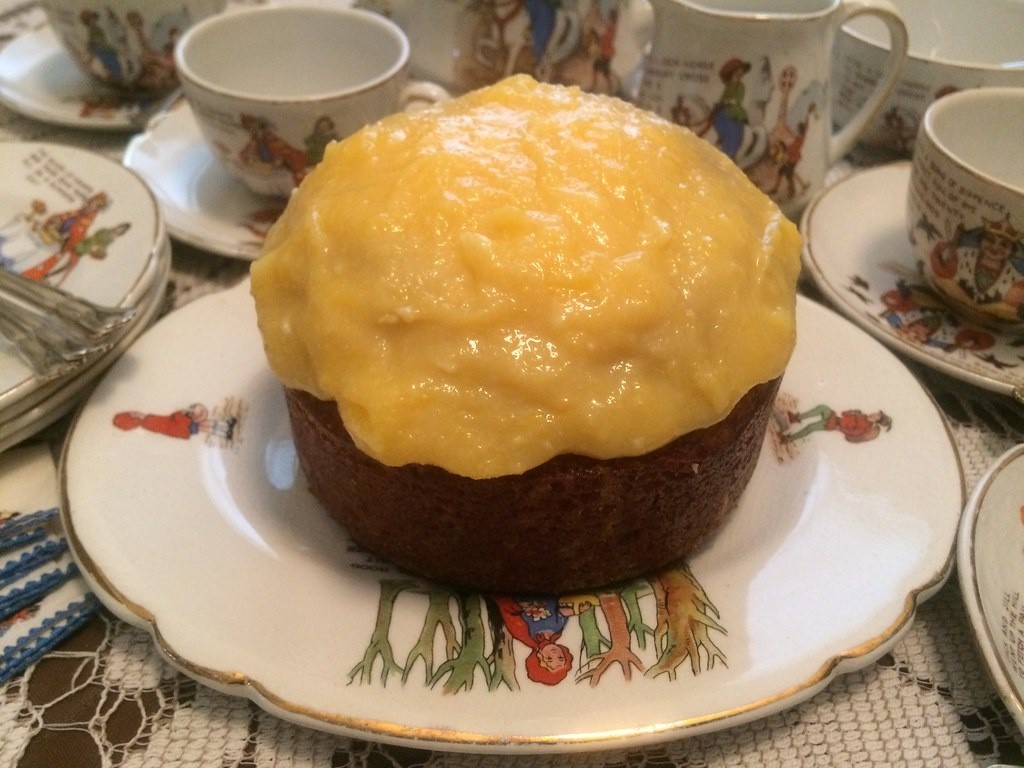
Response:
[[800, 161, 1024, 395], [121, 89, 433, 260], [0, 143, 174, 455], [955, 445, 1024, 735], [56, 275, 965, 755], [0, 24, 151, 128]]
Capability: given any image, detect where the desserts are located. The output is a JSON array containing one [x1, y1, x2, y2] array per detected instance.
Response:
[[250, 71, 800, 598]]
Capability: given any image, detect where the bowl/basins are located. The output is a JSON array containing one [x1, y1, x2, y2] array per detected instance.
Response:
[[835, 1, 1023, 152]]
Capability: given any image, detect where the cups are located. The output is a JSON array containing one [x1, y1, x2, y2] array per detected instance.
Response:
[[171, 3, 451, 198], [903, 90, 1024, 328], [390, 1, 646, 105], [43, 0, 228, 93]]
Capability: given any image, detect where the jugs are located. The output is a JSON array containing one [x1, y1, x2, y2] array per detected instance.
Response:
[[636, 0, 911, 220]]
